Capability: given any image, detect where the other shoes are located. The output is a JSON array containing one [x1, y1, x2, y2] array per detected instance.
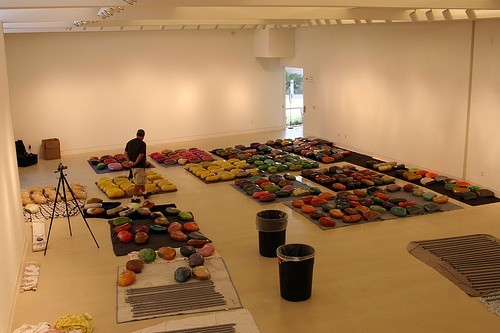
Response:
[[131, 199, 140, 203], [143, 192, 152, 199]]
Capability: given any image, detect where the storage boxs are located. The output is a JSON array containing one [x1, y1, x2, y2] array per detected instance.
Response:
[[42, 138, 62, 160]]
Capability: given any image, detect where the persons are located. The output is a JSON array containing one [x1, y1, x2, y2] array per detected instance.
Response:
[[124, 129, 151, 203]]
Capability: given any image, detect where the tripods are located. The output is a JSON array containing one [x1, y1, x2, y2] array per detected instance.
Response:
[[44, 172, 101, 254]]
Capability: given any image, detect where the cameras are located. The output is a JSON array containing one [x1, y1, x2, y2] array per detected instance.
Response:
[[57, 163, 67, 171]]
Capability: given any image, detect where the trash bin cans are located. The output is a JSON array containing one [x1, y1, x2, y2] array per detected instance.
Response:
[[276, 243, 316, 302], [255, 209, 289, 258]]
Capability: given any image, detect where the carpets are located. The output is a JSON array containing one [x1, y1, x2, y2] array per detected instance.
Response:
[[87, 158, 155, 173], [407, 233, 500, 316], [229, 175, 322, 205], [284, 185, 464, 230], [83, 199, 180, 218], [117, 256, 243, 322], [208, 136, 372, 174], [95, 174, 178, 199], [22, 185, 88, 217], [300, 165, 396, 191], [108, 211, 213, 255], [345, 156, 500, 206], [184, 161, 266, 184], [127, 246, 222, 262], [147, 147, 217, 167], [132, 307, 260, 332]]
